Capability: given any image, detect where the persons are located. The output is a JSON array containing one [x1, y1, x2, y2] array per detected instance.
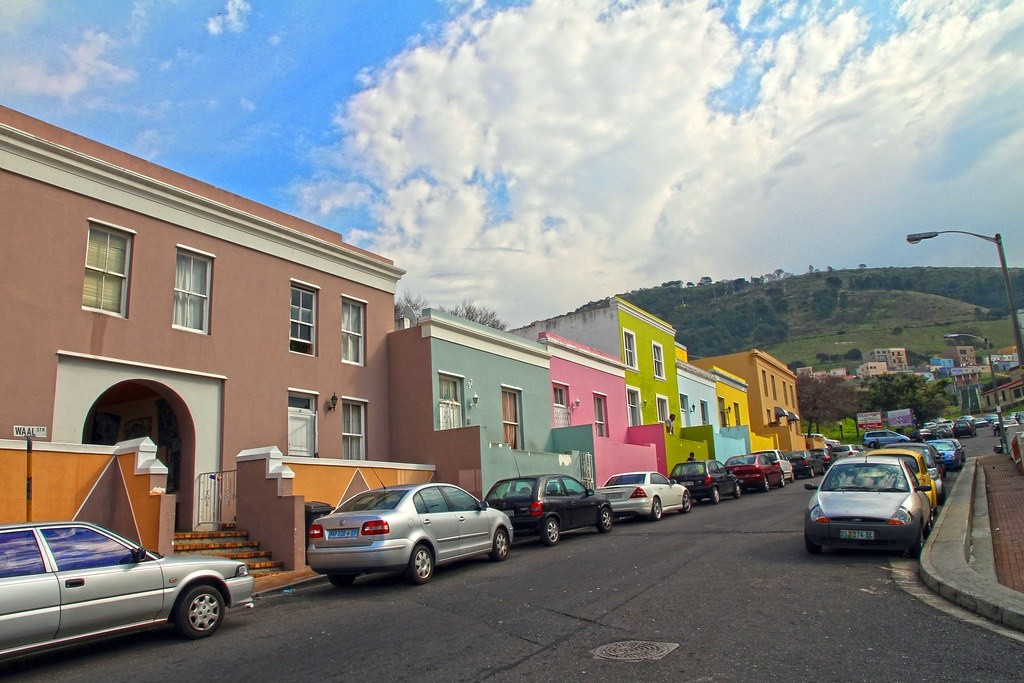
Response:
[[686, 452, 695, 461], [665, 413, 675, 434]]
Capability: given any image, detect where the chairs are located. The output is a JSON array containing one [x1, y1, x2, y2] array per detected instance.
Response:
[[683, 467, 699, 473], [546, 484, 558, 496], [521, 487, 530, 494]]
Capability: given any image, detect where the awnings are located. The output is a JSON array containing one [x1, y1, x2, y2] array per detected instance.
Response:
[[776, 407, 788, 417], [787, 411, 799, 422]]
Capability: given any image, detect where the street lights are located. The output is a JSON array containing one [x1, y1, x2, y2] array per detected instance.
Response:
[[907, 231, 1024, 384], [943, 334, 1007, 454]]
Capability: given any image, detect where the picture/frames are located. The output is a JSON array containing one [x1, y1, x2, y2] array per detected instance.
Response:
[[122, 416, 152, 441]]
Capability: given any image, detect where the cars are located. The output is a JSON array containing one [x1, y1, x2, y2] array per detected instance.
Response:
[[595, 472, 691, 521], [801, 433, 841, 452], [927, 439, 967, 472], [785, 450, 825, 480], [811, 449, 834, 471], [724, 455, 784, 493], [885, 443, 946, 506], [909, 411, 1024, 443], [669, 460, 741, 505], [865, 448, 937, 528], [485, 474, 613, 546], [750, 450, 794, 485], [804, 456, 933, 561], [831, 444, 866, 460], [862, 430, 912, 449], [307, 482, 514, 588], [0, 522, 255, 661]]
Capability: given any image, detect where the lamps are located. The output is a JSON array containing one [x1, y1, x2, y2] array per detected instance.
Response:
[[329, 392, 339, 412], [470, 393, 479, 409], [727, 406, 731, 414], [572, 398, 580, 411], [690, 404, 696, 412], [641, 400, 647, 409]]
